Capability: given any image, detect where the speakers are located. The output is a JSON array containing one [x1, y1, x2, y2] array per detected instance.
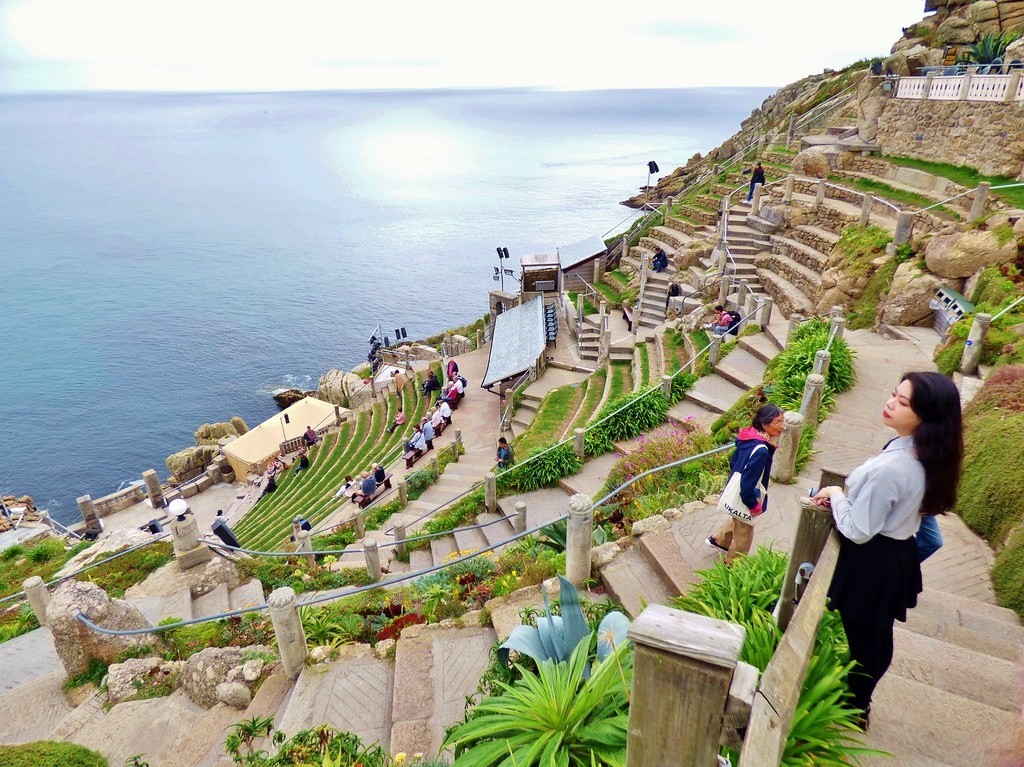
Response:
[[384, 327, 407, 346], [497, 247, 509, 258]]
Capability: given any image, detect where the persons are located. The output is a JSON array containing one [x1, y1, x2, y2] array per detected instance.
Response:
[[331, 463, 385, 496], [452, 372, 467, 388], [447, 356, 459, 381], [254, 425, 318, 499], [706, 405, 784, 570], [386, 407, 405, 432], [809, 372, 964, 735], [710, 306, 734, 334], [666, 284, 678, 310], [442, 382, 458, 402], [419, 371, 440, 398], [215, 509, 230, 526], [429, 406, 442, 428], [420, 417, 434, 442], [495, 438, 514, 468], [453, 376, 464, 395], [407, 425, 425, 451], [649, 247, 668, 273], [743, 162, 765, 203], [290, 514, 312, 535], [438, 400, 452, 417]]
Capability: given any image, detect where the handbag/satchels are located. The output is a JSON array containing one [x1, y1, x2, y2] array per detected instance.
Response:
[[673, 284, 683, 295], [718, 444, 770, 527]]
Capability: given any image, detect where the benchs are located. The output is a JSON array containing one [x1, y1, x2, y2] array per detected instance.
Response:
[[449, 393, 463, 410], [354, 473, 393, 509], [402, 435, 436, 468], [434, 411, 453, 437]]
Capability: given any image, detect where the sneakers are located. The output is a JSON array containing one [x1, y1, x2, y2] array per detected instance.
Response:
[[706, 536, 730, 553]]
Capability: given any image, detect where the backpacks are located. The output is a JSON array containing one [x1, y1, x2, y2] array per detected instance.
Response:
[[458, 375, 467, 387], [722, 310, 741, 336]]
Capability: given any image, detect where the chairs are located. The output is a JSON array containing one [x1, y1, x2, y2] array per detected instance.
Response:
[[921, 58, 1024, 96]]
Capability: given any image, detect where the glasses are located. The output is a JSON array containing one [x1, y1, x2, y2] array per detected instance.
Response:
[[769, 419, 786, 426]]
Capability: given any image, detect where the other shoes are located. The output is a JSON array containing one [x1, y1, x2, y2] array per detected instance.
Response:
[[845, 707, 869, 732]]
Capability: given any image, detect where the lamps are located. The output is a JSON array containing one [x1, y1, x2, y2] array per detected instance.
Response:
[[169, 498, 187, 521]]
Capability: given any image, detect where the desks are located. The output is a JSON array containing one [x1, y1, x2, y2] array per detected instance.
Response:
[[915, 63, 1024, 70]]
